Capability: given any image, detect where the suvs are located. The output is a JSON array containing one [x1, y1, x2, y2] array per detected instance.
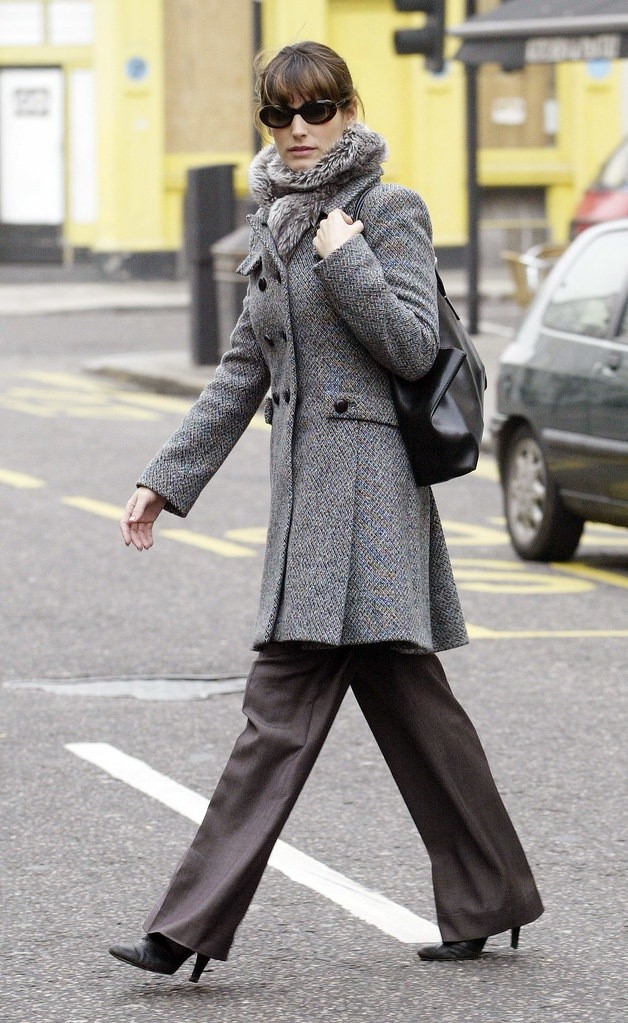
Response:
[[486, 217, 628, 562]]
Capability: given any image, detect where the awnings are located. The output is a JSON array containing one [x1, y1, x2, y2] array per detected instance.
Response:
[[455, 1, 628, 70]]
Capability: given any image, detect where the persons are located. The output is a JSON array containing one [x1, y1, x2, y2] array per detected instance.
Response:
[[109, 43, 545, 983]]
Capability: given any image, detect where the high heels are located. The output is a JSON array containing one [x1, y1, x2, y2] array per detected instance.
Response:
[[418, 925, 520, 961], [107, 930, 211, 983]]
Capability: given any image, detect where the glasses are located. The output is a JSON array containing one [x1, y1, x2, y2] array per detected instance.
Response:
[[259, 97, 349, 128]]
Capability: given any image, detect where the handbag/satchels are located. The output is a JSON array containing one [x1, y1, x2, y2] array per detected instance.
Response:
[[352, 180, 491, 487]]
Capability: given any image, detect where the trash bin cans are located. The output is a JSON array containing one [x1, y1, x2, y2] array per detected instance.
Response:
[[209, 226, 253, 359]]
